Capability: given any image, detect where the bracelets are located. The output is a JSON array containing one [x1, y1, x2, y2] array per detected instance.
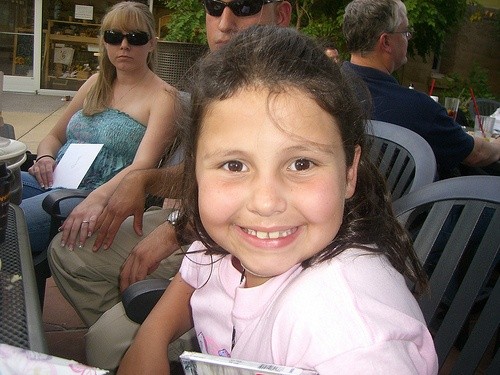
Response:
[[34, 155, 56, 163]]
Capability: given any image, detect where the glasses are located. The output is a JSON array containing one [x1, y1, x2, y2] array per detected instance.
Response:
[[198, 0, 282, 17], [103, 30, 150, 46], [376, 30, 416, 41]]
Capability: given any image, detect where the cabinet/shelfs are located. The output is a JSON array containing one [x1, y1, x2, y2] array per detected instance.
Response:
[[45, 20, 101, 88]]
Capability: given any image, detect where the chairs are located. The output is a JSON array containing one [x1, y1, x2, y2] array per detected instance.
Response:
[[20, 98, 500, 375]]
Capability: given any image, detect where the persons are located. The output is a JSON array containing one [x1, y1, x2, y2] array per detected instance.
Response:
[[339, 1, 500, 181], [1, 2, 184, 272], [46, 1, 293, 370], [117, 25, 438, 375], [320, 43, 339, 66]]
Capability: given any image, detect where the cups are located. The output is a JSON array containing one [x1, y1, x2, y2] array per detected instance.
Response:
[[474, 115, 495, 144], [444, 96, 460, 123]]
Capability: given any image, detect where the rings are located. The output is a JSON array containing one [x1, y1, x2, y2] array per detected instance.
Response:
[[82, 221, 88, 224]]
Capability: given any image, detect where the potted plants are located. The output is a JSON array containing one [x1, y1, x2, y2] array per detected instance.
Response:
[[154, 0, 209, 87]]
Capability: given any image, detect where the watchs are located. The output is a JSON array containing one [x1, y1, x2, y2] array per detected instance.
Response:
[[166, 210, 180, 226]]
